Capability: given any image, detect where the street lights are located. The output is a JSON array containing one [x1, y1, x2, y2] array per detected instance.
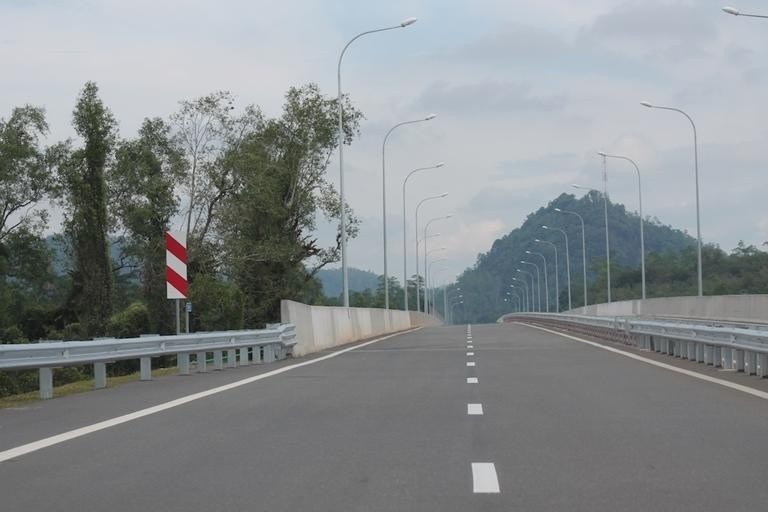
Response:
[[380, 113, 438, 307], [640, 101, 707, 297], [402, 159, 464, 325], [570, 183, 613, 305], [336, 15, 417, 310], [503, 206, 588, 311], [597, 151, 647, 298]]
